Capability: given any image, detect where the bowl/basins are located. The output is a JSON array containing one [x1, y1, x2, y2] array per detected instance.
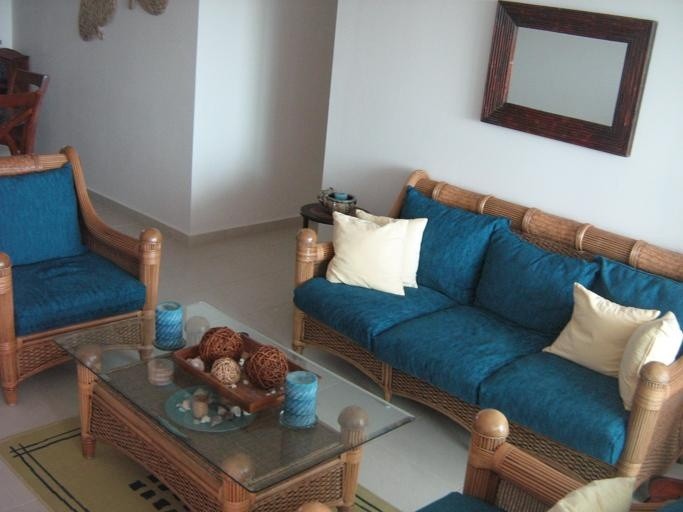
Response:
[[322, 192, 356, 214]]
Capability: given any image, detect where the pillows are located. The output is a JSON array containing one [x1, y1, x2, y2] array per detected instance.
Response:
[[611, 309, 683, 412], [396, 183, 512, 314], [590, 253, 683, 364], [353, 208, 428, 289], [323, 210, 409, 297], [539, 280, 662, 382], [471, 218, 601, 340], [545, 475, 638, 512]]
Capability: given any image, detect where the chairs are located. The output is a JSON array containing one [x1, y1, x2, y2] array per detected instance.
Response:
[[0, 45, 51, 157], [292, 407, 683, 512]]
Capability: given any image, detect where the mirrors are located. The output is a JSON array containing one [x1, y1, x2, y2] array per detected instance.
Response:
[[477, 0, 659, 159]]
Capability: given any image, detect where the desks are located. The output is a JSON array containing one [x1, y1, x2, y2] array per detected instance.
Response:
[[296, 201, 371, 232]]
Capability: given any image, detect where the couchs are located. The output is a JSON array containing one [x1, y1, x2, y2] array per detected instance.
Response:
[[286, 163, 683, 504], [0, 145, 166, 408]]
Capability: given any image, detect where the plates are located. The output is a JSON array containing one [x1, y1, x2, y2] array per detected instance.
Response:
[[165, 385, 258, 431]]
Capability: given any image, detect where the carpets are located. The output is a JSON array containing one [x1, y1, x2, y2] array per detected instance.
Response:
[[0, 409, 409, 511]]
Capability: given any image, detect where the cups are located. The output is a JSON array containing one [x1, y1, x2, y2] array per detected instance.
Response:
[[155, 300, 183, 349], [283, 369, 317, 428], [146, 359, 174, 386]]
